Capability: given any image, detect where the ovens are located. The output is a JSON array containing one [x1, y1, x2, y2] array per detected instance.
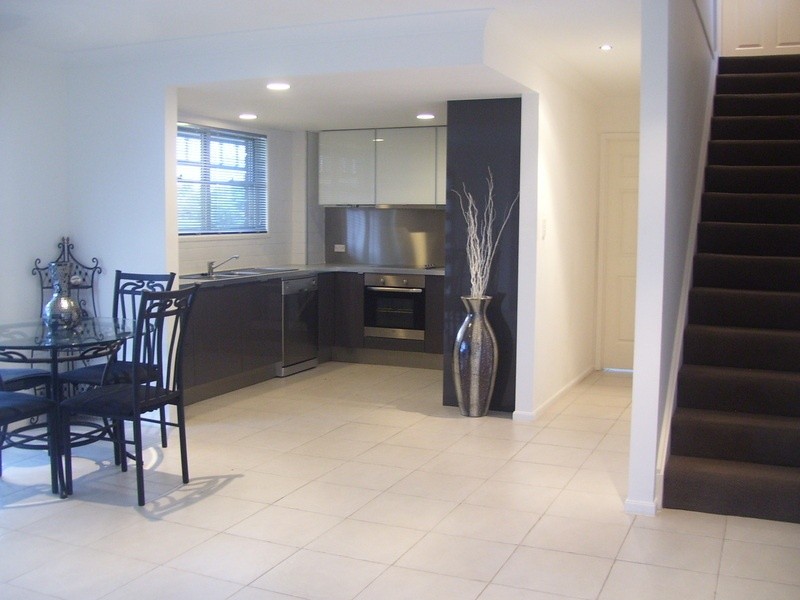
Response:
[[363, 272, 425, 342]]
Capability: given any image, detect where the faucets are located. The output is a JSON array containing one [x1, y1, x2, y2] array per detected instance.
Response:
[[206, 255, 240, 275]]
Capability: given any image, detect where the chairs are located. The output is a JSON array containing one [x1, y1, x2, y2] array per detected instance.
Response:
[[58, 280, 197, 505], [0, 369, 55, 458], [55, 269, 178, 465], [0, 376, 68, 501]]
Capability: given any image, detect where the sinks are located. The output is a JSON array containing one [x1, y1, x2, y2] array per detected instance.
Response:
[[227, 265, 299, 275], [179, 274, 243, 282]]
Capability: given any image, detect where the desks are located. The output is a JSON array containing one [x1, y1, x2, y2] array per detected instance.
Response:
[[1, 316, 158, 496]]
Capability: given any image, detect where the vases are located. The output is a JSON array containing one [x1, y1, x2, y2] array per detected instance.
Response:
[[451, 164, 520, 416]]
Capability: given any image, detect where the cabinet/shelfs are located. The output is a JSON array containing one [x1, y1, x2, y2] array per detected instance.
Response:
[[318, 128, 446, 215], [420, 274, 446, 353], [177, 281, 280, 406], [320, 271, 364, 353]]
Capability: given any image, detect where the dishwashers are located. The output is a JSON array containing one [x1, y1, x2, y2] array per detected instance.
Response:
[[275, 271, 320, 378]]
